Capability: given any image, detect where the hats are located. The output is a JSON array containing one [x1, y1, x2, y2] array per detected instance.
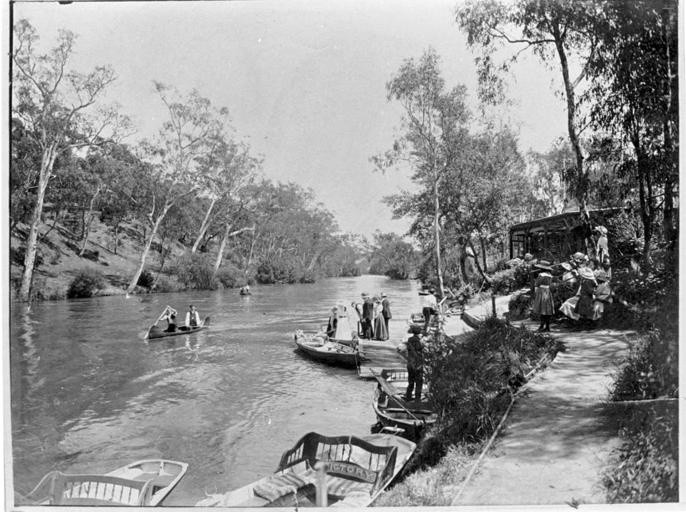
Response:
[[362, 291, 387, 304], [534, 260, 553, 270], [594, 226, 608, 236], [331, 306, 338, 313], [560, 252, 608, 282], [408, 325, 422, 334]]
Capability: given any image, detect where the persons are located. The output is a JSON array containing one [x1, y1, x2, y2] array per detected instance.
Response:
[[158, 305, 178, 333], [240, 283, 249, 292], [404, 324, 425, 404], [523, 226, 611, 333], [184, 305, 199, 331], [328, 292, 392, 341]]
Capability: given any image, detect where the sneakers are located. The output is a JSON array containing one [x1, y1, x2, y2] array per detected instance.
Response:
[[535, 327, 549, 333]]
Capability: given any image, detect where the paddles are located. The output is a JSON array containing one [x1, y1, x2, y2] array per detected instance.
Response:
[[144, 305, 171, 340]]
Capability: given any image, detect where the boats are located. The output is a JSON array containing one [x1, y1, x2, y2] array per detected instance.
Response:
[[240, 288, 251, 295], [372, 369, 437, 443], [294, 324, 361, 369], [148, 317, 211, 339]]
